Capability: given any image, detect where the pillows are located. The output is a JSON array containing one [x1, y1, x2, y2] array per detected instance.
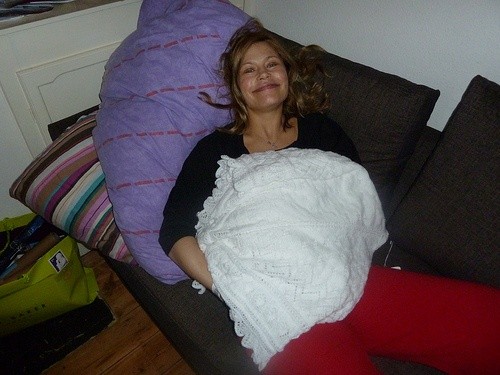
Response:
[[9, 114, 139, 268], [297, 46, 440, 209], [388, 75, 500, 288]]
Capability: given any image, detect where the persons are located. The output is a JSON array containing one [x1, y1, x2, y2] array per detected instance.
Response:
[[159, 19, 500, 375]]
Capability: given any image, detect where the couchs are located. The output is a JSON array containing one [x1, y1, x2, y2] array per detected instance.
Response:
[[47, 24, 500, 375]]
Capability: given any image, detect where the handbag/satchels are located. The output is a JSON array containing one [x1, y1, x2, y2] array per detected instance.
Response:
[[0, 214, 99, 331]]
